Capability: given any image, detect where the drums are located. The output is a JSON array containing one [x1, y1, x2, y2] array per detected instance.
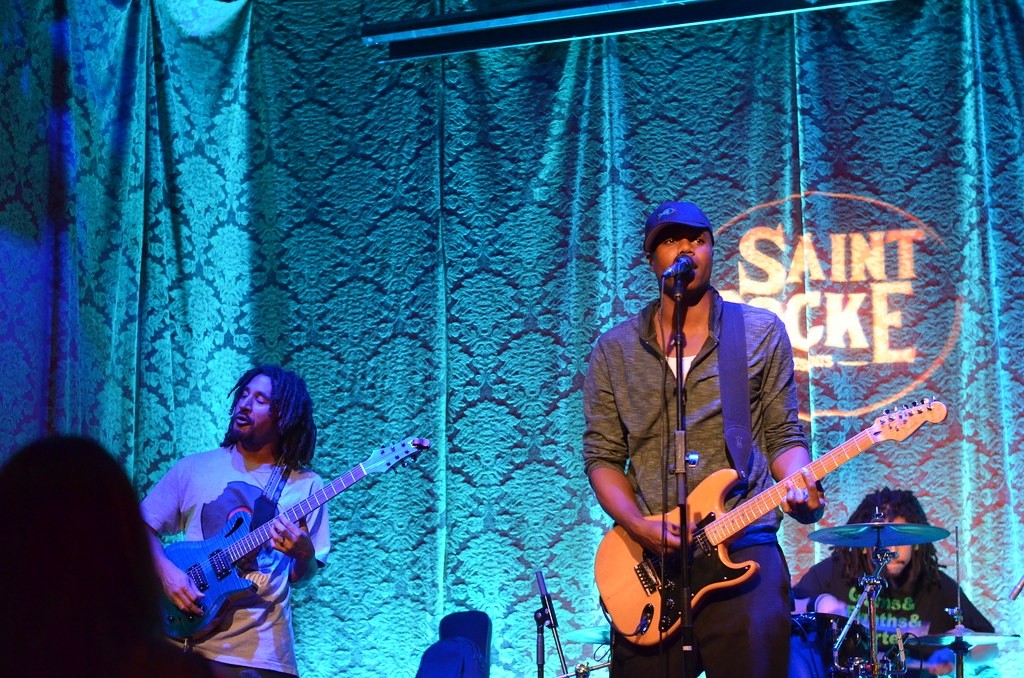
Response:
[[788, 612, 878, 678]]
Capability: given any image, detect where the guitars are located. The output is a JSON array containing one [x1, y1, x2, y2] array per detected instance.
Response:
[[594, 396, 948, 647], [158, 434, 431, 642]]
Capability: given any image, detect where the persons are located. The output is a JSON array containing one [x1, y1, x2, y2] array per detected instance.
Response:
[[582, 202, 824, 678], [786, 488, 999, 678], [0, 433, 220, 678], [136, 365, 330, 678]]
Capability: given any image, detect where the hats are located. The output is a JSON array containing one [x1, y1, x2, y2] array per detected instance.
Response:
[[643, 202, 714, 255]]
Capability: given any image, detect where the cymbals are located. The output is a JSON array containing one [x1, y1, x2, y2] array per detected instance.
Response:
[[807, 523, 951, 548], [904, 628, 1020, 646]]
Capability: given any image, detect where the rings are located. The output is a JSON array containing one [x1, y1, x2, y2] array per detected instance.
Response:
[[279, 537, 285, 544]]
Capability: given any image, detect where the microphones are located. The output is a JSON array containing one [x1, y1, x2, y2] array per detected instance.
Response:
[[1009, 576, 1024, 600], [880, 632, 909, 665], [661, 253, 694, 280]]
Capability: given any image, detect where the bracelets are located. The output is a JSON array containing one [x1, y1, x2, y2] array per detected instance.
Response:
[[806, 591, 833, 612]]
